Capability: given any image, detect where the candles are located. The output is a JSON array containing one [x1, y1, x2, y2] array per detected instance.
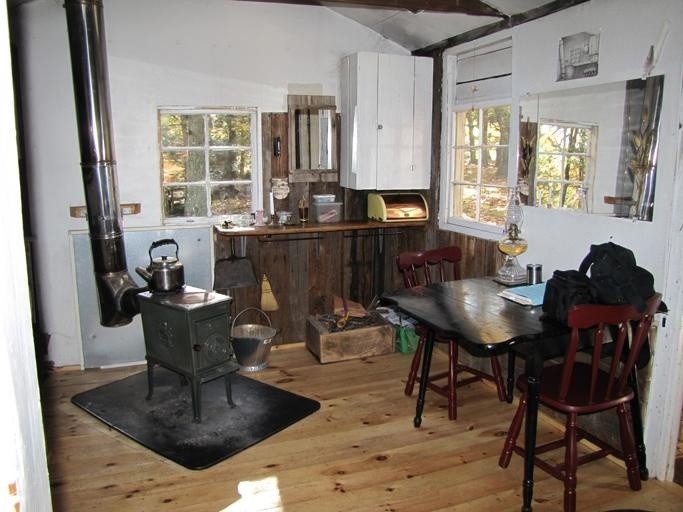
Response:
[[269, 192, 275, 214]]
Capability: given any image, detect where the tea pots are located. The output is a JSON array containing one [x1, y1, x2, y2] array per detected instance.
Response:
[[135, 239, 184, 292]]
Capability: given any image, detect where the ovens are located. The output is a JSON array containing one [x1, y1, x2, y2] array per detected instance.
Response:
[[367, 192, 429, 222]]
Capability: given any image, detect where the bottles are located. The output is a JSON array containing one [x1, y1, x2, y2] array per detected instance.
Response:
[[299, 195, 309, 222], [253, 208, 265, 226]]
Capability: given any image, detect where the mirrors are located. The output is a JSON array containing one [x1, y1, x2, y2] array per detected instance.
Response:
[[515, 75, 665, 221]]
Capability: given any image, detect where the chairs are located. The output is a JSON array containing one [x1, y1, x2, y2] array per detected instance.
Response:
[[396, 246, 507, 420], [498, 294, 664, 511]]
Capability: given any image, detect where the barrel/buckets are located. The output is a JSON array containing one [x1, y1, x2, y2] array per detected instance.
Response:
[[231, 307, 277, 371]]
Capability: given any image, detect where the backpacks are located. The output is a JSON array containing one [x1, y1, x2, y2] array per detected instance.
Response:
[[578, 243, 668, 369]]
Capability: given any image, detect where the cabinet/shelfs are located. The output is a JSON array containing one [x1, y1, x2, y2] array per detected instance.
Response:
[[340, 52, 434, 190]]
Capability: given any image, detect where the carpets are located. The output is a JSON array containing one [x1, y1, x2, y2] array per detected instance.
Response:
[[70, 364, 320, 470]]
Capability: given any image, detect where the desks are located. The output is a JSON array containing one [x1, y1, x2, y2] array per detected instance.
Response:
[[379, 275, 649, 511]]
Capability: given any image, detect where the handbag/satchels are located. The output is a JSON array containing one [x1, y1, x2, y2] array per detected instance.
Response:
[[540, 269, 599, 329]]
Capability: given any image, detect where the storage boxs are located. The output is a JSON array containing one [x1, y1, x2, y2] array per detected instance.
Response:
[[304, 314, 395, 364], [313, 202, 343, 223]]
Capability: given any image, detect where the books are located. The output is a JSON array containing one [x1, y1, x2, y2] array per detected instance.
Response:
[[497, 282, 546, 307], [334, 295, 368, 318]]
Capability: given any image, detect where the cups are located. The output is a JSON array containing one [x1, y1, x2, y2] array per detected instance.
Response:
[[526, 263, 543, 285]]
